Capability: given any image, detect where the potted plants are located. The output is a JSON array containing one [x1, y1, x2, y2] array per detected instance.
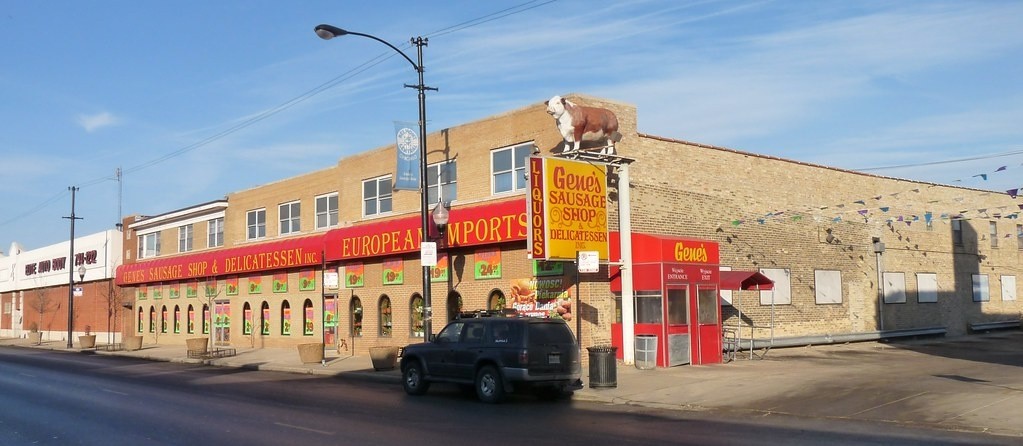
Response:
[[379, 299, 392, 337], [488, 293, 507, 319], [411, 297, 426, 338], [351, 302, 364, 337], [27, 322, 43, 346], [77, 325, 96, 349]]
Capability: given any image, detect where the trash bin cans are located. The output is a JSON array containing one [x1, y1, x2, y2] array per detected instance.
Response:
[[634, 334, 658, 370], [586, 346, 618, 388]]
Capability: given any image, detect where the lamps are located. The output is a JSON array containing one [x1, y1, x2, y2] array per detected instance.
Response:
[[825, 229, 835, 244], [75, 253, 92, 282]]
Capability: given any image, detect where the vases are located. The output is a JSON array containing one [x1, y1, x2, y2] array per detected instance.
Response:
[[122, 335, 143, 352], [186, 337, 209, 358], [369, 345, 399, 372], [301, 344, 325, 365]]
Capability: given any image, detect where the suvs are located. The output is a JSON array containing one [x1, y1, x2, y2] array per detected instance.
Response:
[[398, 308, 585, 405]]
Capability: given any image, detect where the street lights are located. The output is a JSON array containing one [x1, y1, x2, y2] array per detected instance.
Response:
[[64, 265, 87, 350], [314, 23, 451, 344]]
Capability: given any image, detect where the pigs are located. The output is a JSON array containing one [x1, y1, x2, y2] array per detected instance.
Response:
[[545, 95, 619, 154]]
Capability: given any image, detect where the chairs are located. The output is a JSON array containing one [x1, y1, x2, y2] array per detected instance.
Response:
[[466, 328, 485, 346]]
